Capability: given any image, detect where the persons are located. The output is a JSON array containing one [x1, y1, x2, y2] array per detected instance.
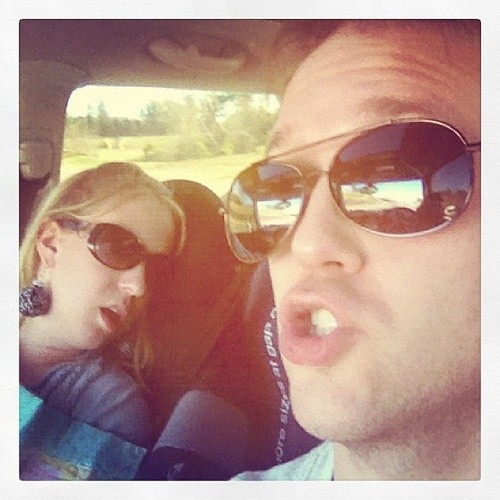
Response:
[[19, 161, 190, 480], [223, 18, 480, 481]]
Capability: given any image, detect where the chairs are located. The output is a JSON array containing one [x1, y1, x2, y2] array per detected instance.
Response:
[[141, 179, 251, 419]]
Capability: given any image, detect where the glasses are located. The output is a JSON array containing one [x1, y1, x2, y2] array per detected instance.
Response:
[[48, 209, 179, 295], [220, 117, 481, 265]]
